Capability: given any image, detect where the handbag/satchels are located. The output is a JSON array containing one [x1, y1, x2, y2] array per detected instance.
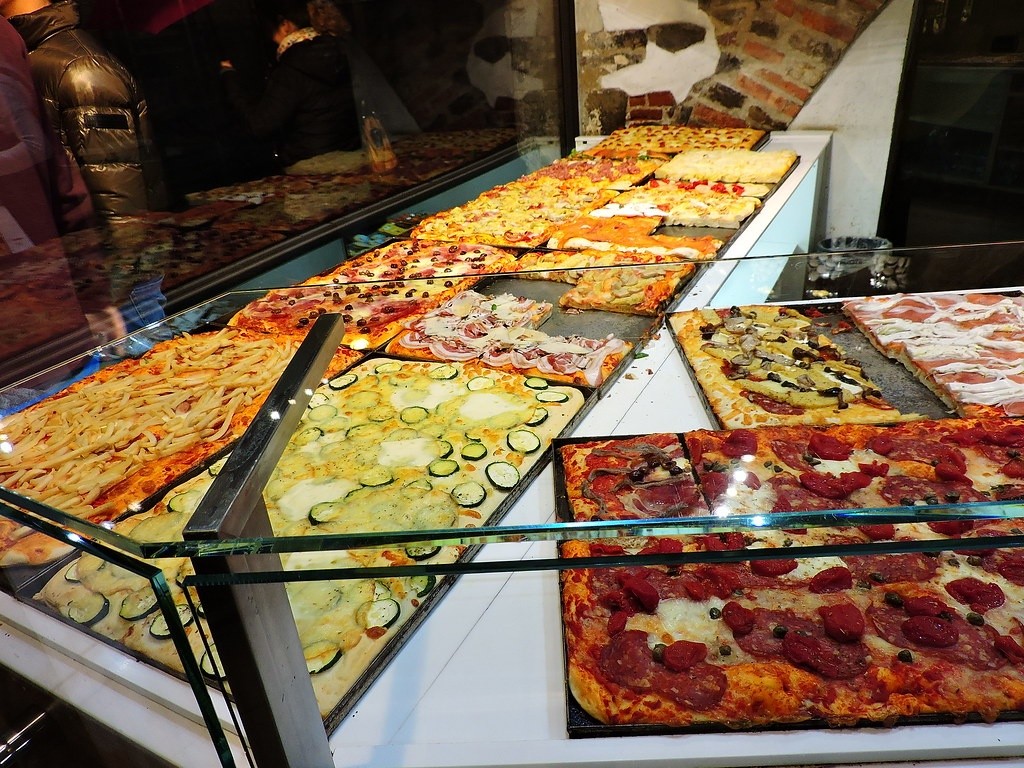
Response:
[[142, 158, 175, 212], [47, 148, 93, 228]]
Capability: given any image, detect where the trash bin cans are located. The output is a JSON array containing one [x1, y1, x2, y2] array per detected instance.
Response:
[[813, 235, 893, 298]]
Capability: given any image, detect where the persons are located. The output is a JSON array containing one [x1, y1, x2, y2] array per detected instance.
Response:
[[0, 0, 171, 418], [220, 12, 361, 166]]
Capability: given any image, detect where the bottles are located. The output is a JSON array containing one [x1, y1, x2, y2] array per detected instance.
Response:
[[361, 97, 398, 175]]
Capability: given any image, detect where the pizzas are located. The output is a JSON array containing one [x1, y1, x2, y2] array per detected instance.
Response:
[[0, 124, 1023, 727]]
